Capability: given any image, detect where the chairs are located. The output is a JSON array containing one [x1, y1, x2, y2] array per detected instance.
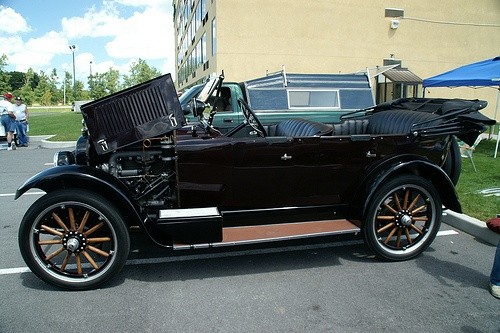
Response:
[[341, 110, 441, 135], [455, 135, 484, 172]]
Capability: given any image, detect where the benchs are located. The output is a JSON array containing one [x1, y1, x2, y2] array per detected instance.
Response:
[[274, 118, 333, 137]]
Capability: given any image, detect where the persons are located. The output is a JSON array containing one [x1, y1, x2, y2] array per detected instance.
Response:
[[14, 97, 28, 147], [0, 93, 16, 150], [489, 238, 500, 298]]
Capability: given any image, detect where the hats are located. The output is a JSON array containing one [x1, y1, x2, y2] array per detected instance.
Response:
[[15, 97, 22, 100], [6, 93, 14, 99]]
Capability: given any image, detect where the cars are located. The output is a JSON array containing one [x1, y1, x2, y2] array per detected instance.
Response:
[[15, 74, 496, 291]]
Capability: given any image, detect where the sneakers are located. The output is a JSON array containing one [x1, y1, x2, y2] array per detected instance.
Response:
[[11, 141, 16, 150], [488, 282, 500, 298], [7, 146, 12, 151]]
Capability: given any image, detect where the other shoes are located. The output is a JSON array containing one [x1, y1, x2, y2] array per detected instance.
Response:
[[23, 144, 28, 147], [18, 144, 23, 147]]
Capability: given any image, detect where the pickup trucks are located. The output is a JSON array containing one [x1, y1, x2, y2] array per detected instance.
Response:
[[174, 64, 380, 134]]
